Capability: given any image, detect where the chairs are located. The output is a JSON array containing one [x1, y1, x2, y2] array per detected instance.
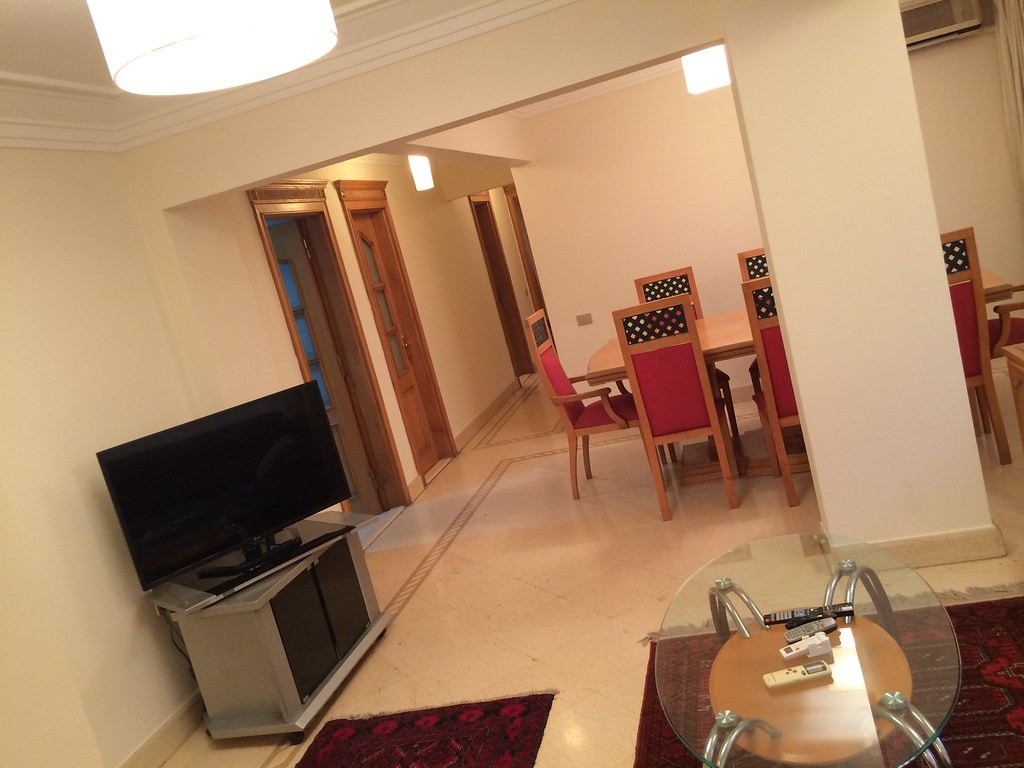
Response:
[[737, 247, 770, 281], [524, 309, 639, 500], [740, 277, 810, 506], [940, 227, 1013, 466], [988, 283, 1024, 359], [634, 266, 741, 464], [612, 293, 737, 521]]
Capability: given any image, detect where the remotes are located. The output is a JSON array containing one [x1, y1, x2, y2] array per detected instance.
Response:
[[763, 602, 854, 689]]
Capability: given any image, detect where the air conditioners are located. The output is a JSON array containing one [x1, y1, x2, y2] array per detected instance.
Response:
[[899, 0, 984, 51]]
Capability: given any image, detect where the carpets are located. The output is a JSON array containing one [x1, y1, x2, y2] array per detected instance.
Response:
[[292, 687, 560, 768], [632, 580, 1024, 768]]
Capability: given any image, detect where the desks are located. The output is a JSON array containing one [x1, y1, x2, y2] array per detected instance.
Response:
[[1001, 343, 1024, 446], [585, 267, 1013, 466]]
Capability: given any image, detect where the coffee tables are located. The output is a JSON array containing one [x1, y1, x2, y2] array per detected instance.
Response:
[[655, 532, 963, 768]]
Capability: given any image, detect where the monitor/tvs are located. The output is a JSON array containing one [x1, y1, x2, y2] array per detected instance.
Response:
[[95, 380, 353, 591]]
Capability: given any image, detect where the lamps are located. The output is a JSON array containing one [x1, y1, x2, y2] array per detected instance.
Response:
[[86, 0, 339, 96]]
